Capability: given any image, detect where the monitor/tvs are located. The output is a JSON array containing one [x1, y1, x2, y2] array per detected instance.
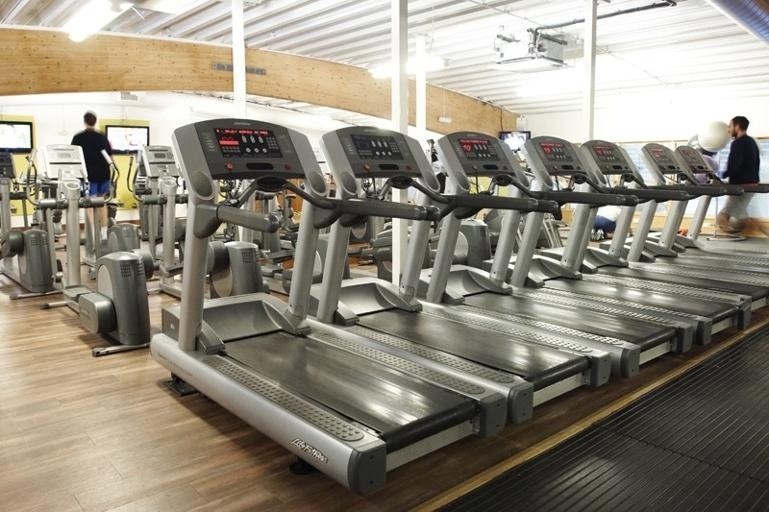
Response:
[[105, 125, 149, 154], [498, 131, 531, 153], [0, 121, 33, 154]]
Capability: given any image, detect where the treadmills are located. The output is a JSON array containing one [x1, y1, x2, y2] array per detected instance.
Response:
[[415, 132, 693, 378], [305, 125, 612, 423], [484, 137, 768, 347], [149, 118, 508, 497]]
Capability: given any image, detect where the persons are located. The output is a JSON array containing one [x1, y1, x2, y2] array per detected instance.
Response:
[[71, 111, 111, 240], [718, 116, 761, 234]]
